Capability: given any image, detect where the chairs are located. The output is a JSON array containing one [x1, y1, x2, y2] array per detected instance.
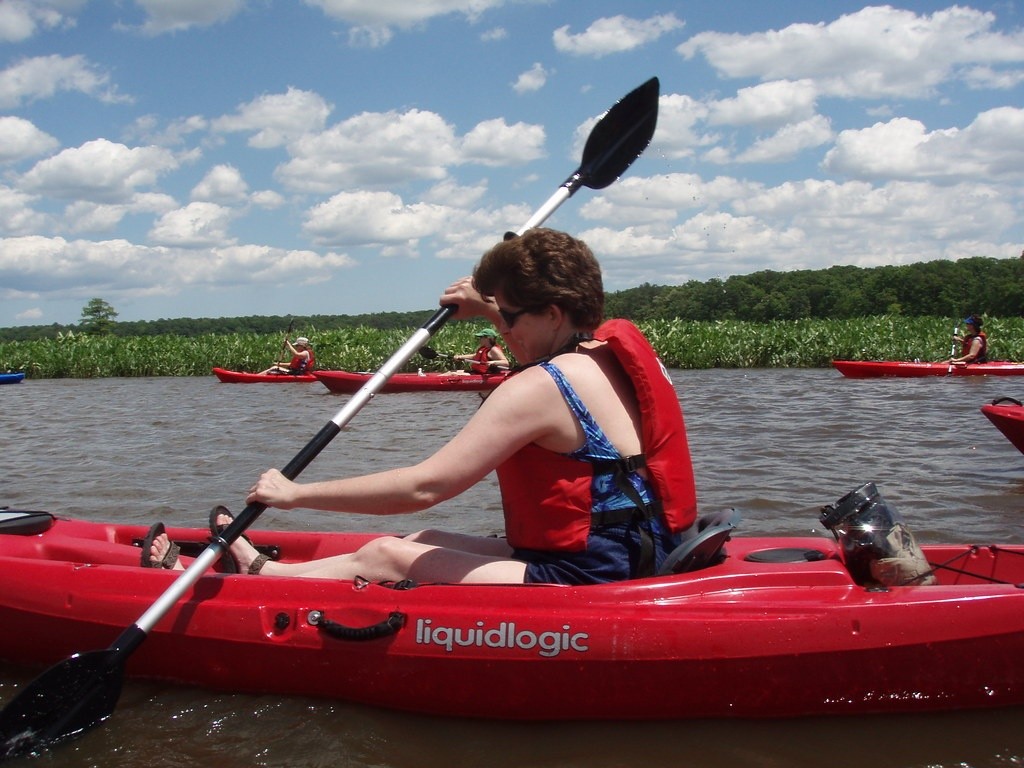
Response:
[[656, 507, 742, 576]]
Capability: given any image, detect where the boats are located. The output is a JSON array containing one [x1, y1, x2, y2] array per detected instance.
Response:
[[832, 358, 1024, 379], [1, 504, 1024, 726], [980, 395, 1024, 458], [0, 372, 25, 386], [311, 369, 514, 394], [212, 366, 316, 384]]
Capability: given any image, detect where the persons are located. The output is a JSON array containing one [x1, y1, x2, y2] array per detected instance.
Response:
[[141, 224, 695, 584], [256, 336, 315, 376], [915, 314, 988, 364], [417, 328, 510, 377]]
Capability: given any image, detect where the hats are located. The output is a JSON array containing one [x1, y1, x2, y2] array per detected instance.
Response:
[[292, 337, 308, 345], [475, 329, 497, 338], [963, 317, 977, 326]]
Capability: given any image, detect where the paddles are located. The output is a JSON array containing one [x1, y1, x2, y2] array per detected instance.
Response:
[[946, 321, 960, 376], [418, 347, 517, 372], [0, 74, 660, 761], [278, 316, 296, 360]]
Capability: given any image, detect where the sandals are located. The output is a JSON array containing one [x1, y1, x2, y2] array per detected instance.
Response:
[[209, 505, 272, 575], [140, 523, 181, 570]]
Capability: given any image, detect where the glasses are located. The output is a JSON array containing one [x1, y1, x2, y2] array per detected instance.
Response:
[[498, 307, 532, 329], [296, 345, 302, 347]]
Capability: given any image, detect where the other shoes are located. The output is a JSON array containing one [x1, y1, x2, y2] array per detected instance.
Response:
[[914, 359, 920, 364], [418, 368, 426, 376]]
